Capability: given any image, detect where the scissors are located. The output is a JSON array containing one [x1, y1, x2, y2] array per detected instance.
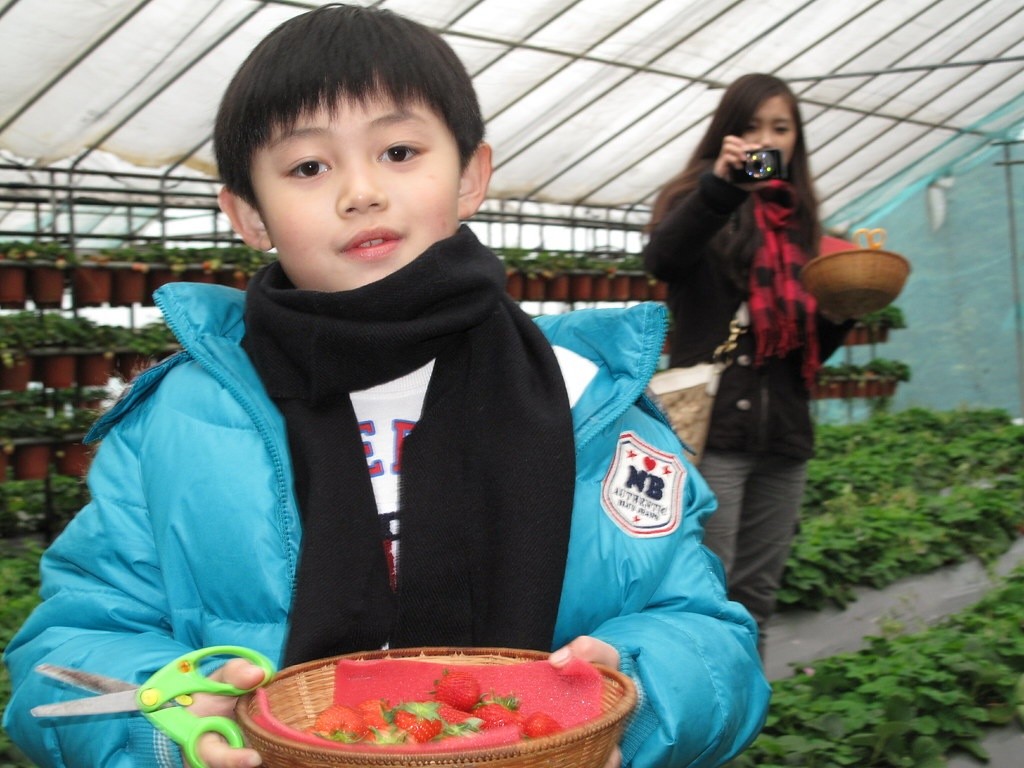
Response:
[[853, 227, 886, 250], [29, 644, 278, 767]]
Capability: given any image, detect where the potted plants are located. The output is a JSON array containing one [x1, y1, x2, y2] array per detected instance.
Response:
[[1, 247, 897, 485]]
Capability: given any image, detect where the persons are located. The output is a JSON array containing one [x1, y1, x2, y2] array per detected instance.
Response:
[[1, 0, 772, 768], [645, 71, 855, 666]]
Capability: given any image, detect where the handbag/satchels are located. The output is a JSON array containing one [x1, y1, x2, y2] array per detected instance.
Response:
[[646, 304, 753, 467]]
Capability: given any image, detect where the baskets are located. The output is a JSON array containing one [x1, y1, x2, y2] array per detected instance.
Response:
[[237, 643, 638, 767], [803, 249, 912, 308]]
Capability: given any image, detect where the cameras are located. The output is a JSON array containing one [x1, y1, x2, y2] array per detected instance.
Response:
[[730, 148, 782, 185]]
